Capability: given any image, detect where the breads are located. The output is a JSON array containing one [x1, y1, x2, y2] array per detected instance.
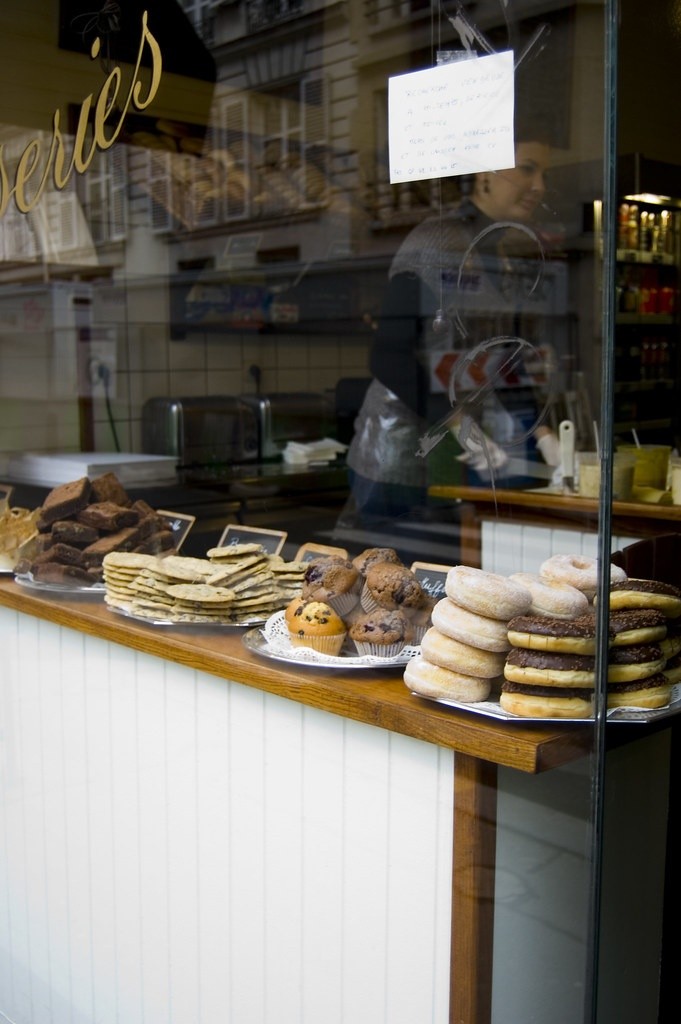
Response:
[[136, 120, 347, 217], [14, 474, 173, 585]]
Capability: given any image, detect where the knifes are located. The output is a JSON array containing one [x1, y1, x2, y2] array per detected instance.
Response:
[[505, 458, 557, 479], [559, 419, 574, 492]]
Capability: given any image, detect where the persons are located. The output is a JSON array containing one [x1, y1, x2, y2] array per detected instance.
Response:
[[344, 45, 570, 567]]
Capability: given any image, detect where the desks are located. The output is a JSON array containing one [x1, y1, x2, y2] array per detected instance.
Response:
[[0, 573, 681, 1024], [427, 484, 681, 572]]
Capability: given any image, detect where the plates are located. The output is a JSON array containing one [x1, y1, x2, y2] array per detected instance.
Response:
[[412, 682, 681, 723], [14, 574, 106, 593], [242, 627, 407, 668], [106, 606, 267, 627], [0, 553, 15, 572]]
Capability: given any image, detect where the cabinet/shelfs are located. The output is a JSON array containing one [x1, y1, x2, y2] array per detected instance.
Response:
[[576, 193, 681, 432]]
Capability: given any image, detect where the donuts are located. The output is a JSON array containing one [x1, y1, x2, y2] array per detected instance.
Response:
[[403, 554, 680, 717]]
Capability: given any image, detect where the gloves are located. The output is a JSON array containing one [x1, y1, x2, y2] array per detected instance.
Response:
[[452, 421, 511, 474], [537, 437, 580, 494]]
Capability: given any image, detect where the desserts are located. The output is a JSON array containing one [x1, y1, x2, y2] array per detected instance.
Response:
[[285, 550, 423, 658]]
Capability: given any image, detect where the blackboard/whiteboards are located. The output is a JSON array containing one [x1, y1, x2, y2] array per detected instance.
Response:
[[295, 543, 350, 563], [156, 509, 197, 551], [218, 524, 289, 557], [410, 561, 449, 600]]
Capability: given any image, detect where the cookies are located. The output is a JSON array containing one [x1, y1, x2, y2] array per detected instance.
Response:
[[102, 544, 307, 620]]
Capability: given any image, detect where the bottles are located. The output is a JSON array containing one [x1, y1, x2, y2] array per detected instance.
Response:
[[267, 279, 299, 323], [616, 203, 675, 254], [631, 339, 671, 382]]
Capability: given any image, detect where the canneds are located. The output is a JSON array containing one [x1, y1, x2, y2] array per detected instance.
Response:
[[638, 286, 676, 314]]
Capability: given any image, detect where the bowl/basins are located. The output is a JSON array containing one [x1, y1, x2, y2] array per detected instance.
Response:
[[668, 459, 681, 505], [616, 444, 670, 490], [578, 451, 634, 502]]
